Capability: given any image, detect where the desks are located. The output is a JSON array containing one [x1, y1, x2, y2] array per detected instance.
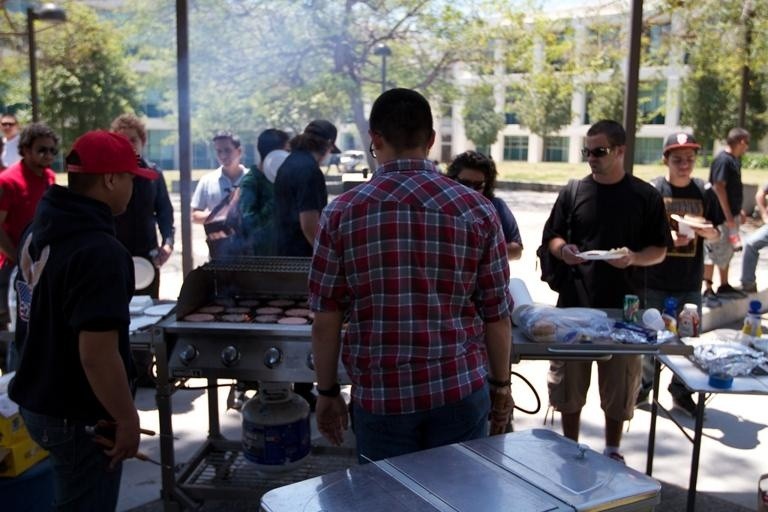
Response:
[[646, 338, 768, 512]]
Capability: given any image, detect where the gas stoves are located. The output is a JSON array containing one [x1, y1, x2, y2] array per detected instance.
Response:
[[153, 291, 349, 376]]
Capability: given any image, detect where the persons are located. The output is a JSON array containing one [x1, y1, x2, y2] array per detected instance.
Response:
[[191, 121, 341, 409], [0, 114, 176, 511], [308, 87, 523, 464], [542, 120, 768, 460]]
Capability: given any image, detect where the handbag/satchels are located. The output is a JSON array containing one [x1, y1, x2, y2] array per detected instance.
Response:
[[202, 218, 241, 258]]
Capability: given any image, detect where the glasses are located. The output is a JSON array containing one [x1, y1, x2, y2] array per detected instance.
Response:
[[581, 146, 618, 158], [32, 146, 58, 157], [370, 139, 378, 158], [456, 175, 487, 191]]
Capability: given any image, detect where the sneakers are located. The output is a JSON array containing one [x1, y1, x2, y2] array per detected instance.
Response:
[[604, 450, 626, 466], [633, 386, 649, 410], [717, 284, 747, 298], [703, 288, 722, 308], [730, 283, 757, 293], [672, 394, 700, 417]]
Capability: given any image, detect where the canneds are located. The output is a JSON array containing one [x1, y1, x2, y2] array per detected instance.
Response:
[[623, 294, 640, 322]]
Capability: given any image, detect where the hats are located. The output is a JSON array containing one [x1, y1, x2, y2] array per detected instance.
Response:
[[263, 149, 293, 184], [662, 132, 701, 155], [304, 119, 341, 154], [64, 129, 161, 180]]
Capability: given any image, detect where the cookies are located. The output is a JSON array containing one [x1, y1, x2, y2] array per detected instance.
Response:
[[183, 297, 316, 324]]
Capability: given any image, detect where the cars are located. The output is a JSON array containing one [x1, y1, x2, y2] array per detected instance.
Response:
[[338, 147, 369, 174]]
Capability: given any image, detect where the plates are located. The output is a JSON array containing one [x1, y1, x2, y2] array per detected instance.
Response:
[[133, 255, 158, 290], [141, 302, 176, 320], [575, 249, 628, 260], [670, 214, 714, 232]]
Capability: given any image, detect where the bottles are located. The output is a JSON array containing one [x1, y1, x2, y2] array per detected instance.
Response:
[[728, 225, 742, 254], [740, 300, 763, 340], [663, 296, 698, 338]]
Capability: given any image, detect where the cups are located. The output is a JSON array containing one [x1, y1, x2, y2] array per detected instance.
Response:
[[641, 307, 666, 329], [707, 350, 737, 389]]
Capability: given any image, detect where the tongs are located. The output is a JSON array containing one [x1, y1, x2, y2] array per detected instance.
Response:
[[83, 416, 182, 470]]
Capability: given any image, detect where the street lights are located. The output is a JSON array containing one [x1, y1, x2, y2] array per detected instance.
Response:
[[373, 43, 393, 96], [25, 3, 67, 124]]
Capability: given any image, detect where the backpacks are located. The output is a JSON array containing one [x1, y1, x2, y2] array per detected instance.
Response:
[[535, 176, 581, 294]]
